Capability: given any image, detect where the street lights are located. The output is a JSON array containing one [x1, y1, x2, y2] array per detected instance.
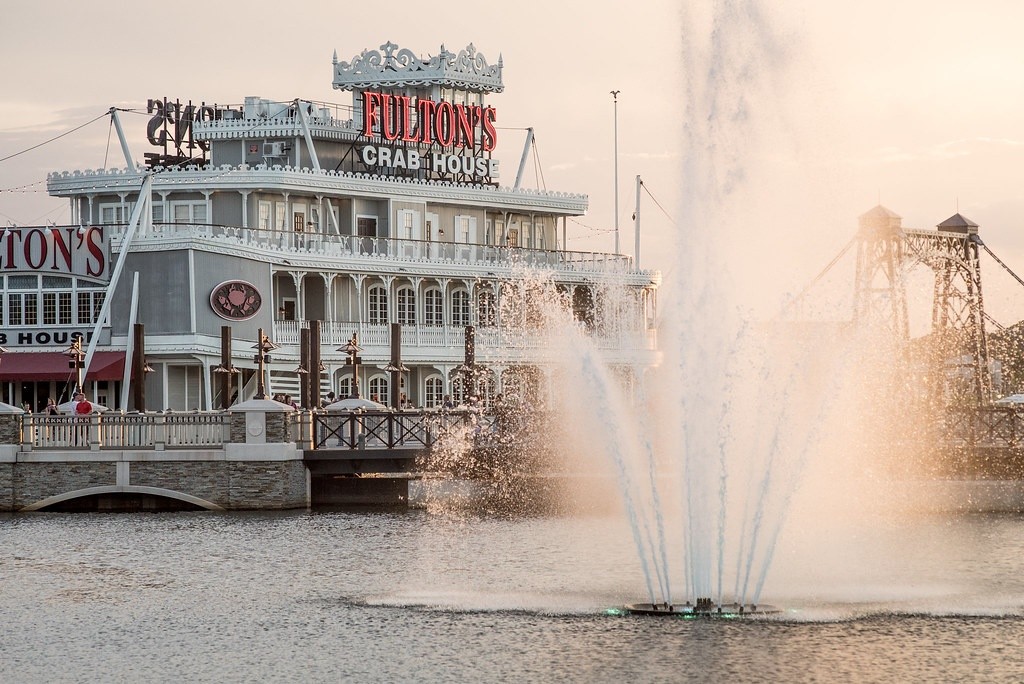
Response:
[[611, 90, 621, 254]]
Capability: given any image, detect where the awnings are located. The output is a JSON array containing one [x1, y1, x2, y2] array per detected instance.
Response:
[[0, 351, 134, 380]]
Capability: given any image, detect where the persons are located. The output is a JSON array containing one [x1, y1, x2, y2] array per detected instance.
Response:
[[441, 395, 453, 408], [400, 393, 415, 409], [321, 392, 381, 409], [272, 393, 296, 411], [472, 393, 510, 407], [21, 403, 32, 443], [70, 392, 92, 447], [45, 398, 59, 442]]
